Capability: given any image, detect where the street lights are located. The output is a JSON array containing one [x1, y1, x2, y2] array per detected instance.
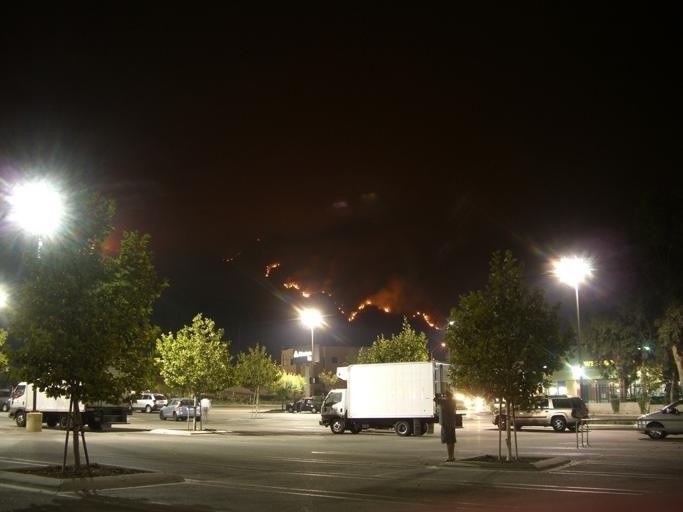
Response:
[[300, 309, 322, 360], [556, 259, 588, 397], [5, 178, 67, 432]]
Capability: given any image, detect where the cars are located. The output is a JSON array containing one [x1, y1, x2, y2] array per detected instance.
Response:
[[0, 389, 10, 412], [632, 400, 683, 440], [130, 392, 201, 421], [492, 394, 588, 432], [286, 397, 317, 413]]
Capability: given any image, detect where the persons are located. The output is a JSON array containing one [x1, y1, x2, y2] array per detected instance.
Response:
[[434, 389, 457, 463], [200, 395, 212, 425]]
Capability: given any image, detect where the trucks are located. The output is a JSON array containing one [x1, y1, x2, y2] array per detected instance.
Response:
[[8, 378, 131, 432], [319, 362, 450, 436]]
